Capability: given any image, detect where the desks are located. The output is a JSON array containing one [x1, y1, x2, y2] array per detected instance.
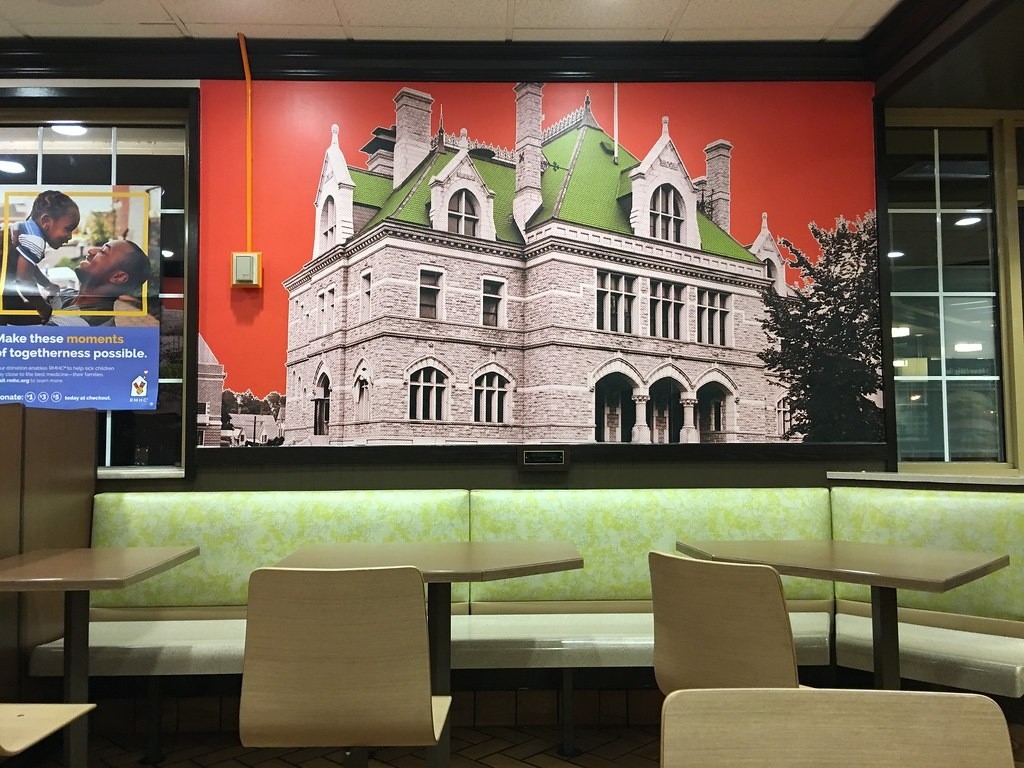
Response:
[[277, 537, 586, 768], [0, 546, 201, 768], [677, 538, 1010, 692]]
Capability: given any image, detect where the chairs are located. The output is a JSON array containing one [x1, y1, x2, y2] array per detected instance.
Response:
[[647, 550, 812, 698], [0, 703, 97, 756], [658, 688, 1015, 768], [239, 565, 453, 748]]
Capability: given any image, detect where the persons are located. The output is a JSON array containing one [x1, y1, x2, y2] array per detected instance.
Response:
[[0, 190, 81, 325], [0, 222, 151, 328]]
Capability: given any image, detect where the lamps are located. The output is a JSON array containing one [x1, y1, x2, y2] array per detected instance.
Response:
[[953, 213, 982, 227]]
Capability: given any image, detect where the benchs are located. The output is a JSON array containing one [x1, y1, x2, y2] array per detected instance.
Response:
[[829, 487, 1024, 726], [30, 486, 836, 759]]
